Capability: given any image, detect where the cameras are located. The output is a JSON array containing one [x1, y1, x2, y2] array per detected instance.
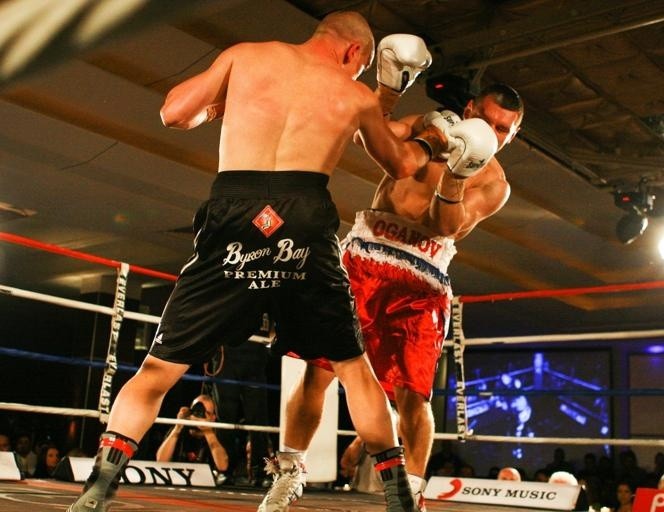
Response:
[[191, 403, 205, 418]]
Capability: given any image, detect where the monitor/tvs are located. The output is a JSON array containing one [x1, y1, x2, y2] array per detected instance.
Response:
[[444, 347, 612, 478]]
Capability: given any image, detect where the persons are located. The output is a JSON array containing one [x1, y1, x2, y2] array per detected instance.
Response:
[[64, 11, 419, 512], [255, 32, 526, 512]]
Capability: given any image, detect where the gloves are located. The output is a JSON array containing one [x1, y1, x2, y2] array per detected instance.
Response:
[[433, 118, 499, 203], [373, 33, 433, 116], [411, 110, 462, 163]]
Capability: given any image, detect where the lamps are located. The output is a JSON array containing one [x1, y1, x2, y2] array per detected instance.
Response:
[[605, 178, 656, 245]]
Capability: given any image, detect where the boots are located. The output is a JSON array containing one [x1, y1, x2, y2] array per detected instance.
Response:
[[64, 430, 139, 512], [256, 449, 308, 512], [406, 473, 426, 512], [370, 446, 421, 512]]
